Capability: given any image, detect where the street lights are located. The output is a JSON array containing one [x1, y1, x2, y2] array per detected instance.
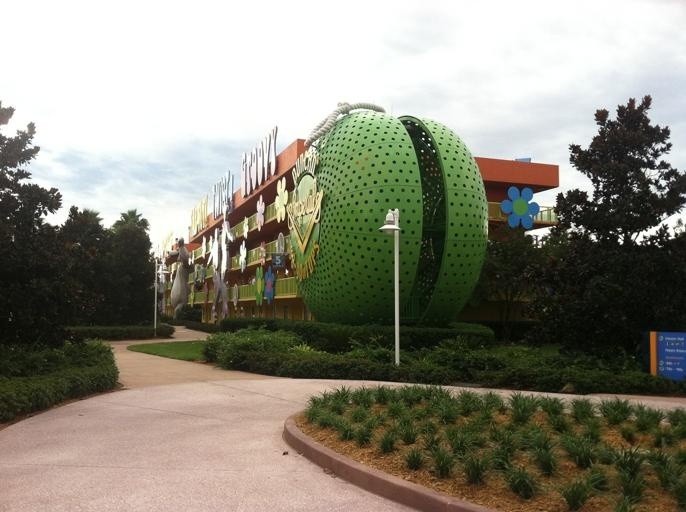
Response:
[[153, 258, 171, 337], [376, 206, 404, 368]]
